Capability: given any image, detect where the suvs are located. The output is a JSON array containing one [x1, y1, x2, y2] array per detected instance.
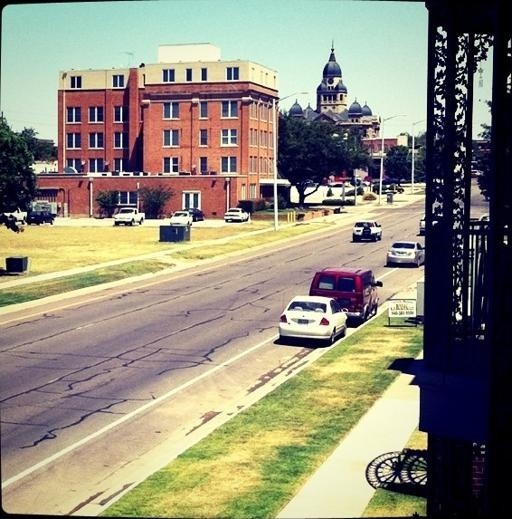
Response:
[[350, 220, 383, 242], [308, 266, 384, 323]]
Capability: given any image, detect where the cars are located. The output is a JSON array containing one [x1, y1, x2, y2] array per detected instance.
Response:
[[26, 209, 55, 226], [298, 177, 371, 187], [183, 208, 205, 222], [169, 210, 195, 227], [385, 240, 425, 268], [277, 294, 348, 346], [471, 170, 483, 177], [224, 207, 249, 222], [419, 214, 425, 236]]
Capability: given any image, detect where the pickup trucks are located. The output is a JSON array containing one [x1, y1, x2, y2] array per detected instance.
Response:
[[113, 207, 146, 227], [3, 207, 27, 225]]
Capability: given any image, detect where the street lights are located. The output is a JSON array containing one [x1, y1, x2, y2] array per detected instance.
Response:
[[272, 90, 310, 229], [411, 118, 426, 192], [378, 113, 410, 204]]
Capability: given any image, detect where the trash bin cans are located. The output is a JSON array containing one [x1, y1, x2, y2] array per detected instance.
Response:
[[387, 193, 394, 203]]
